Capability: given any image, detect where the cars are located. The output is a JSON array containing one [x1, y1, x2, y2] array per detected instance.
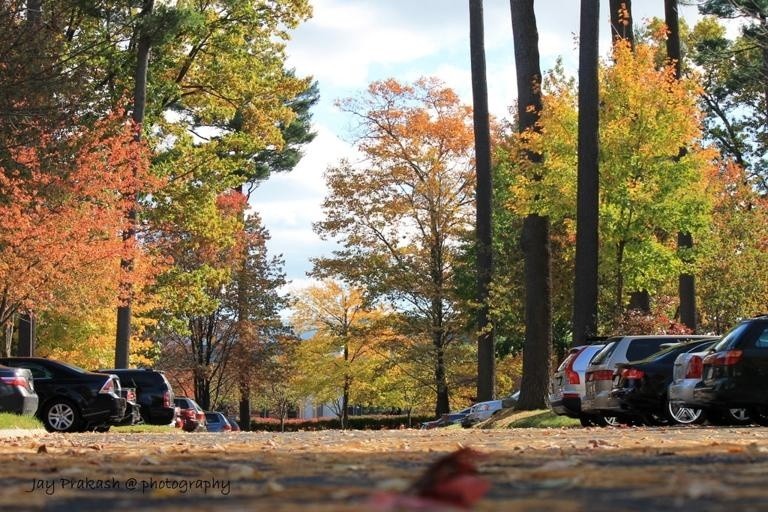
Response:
[[548, 314, 767, 427], [419, 389, 521, 430], [0, 357, 240, 433]]
